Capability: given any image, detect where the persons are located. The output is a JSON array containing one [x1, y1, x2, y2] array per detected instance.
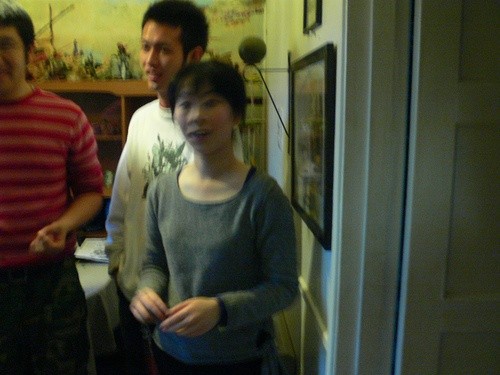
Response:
[[104, 0, 245, 375], [0, 0, 105, 375], [131, 59, 298, 375]]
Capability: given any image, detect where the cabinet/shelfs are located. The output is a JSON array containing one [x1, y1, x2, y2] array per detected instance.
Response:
[[27, 80, 266, 237]]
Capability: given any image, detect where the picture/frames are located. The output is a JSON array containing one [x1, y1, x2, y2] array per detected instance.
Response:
[[288, 42, 336, 251], [303, 0, 322, 34]]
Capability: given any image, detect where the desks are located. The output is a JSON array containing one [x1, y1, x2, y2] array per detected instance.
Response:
[[74, 259, 120, 375]]
